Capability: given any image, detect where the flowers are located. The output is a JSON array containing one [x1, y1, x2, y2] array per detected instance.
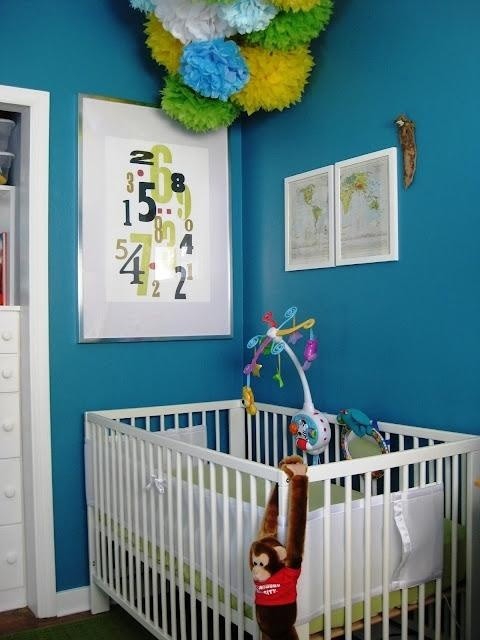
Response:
[[129, 0, 334, 134]]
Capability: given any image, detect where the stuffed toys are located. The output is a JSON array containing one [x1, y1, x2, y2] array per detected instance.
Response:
[[249, 454, 308, 640]]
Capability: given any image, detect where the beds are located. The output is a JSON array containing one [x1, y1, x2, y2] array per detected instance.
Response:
[[82, 400, 480, 639]]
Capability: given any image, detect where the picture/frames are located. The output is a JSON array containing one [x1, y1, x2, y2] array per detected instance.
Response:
[[78, 91, 233, 345], [280, 144, 401, 273]]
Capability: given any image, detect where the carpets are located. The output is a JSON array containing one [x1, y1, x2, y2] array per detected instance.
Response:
[[0, 611, 156, 640]]
